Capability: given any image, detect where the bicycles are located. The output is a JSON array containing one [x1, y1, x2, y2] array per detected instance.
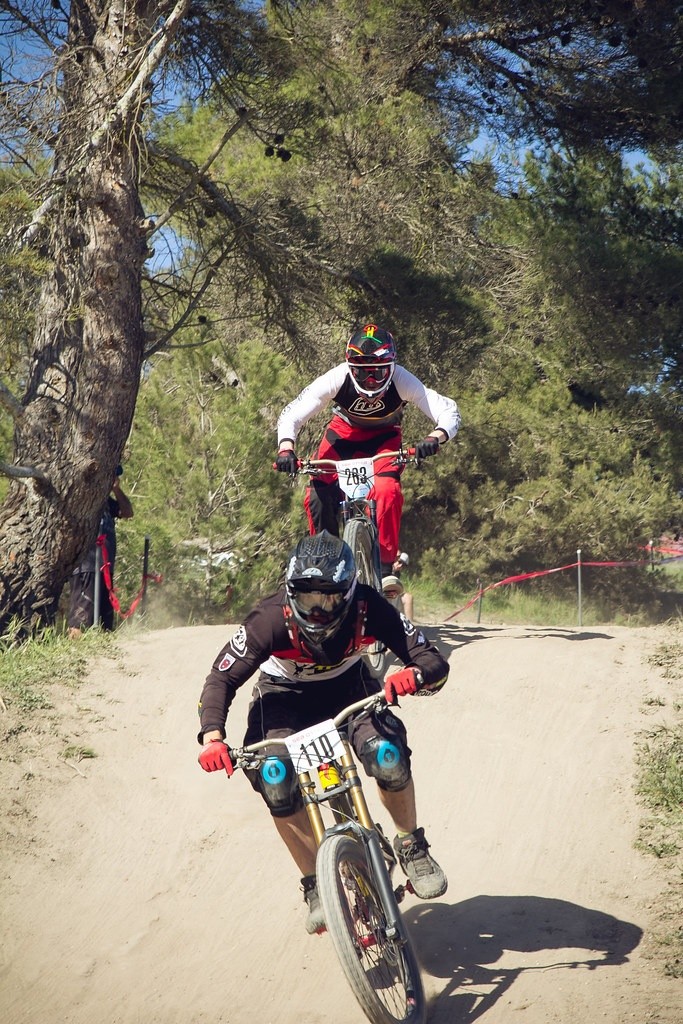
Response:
[[274, 446, 443, 683], [228, 672, 427, 1024]]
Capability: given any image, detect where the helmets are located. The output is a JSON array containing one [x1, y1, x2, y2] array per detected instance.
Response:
[[345, 324, 397, 398], [284, 528, 356, 644]]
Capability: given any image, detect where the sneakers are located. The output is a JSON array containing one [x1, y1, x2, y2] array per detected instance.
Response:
[[299, 874, 325, 935], [393, 827, 448, 900]]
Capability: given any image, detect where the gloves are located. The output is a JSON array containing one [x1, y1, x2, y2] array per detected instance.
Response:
[[385, 667, 424, 703], [276, 450, 299, 478], [415, 435, 438, 465], [198, 739, 237, 776]]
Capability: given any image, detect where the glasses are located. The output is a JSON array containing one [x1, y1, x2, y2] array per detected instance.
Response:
[[291, 588, 347, 618], [351, 367, 389, 384], [398, 561, 404, 567]]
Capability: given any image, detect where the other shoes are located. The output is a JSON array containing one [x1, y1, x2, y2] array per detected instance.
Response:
[[381, 574, 403, 602]]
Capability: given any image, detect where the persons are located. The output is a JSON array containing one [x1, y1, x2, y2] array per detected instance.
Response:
[[276, 324, 460, 593], [391, 546, 414, 621], [67, 476, 134, 639], [196, 528, 450, 934]]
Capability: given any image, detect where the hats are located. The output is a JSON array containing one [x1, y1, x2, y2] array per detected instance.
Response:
[[116, 465, 124, 477], [398, 553, 409, 566]]
